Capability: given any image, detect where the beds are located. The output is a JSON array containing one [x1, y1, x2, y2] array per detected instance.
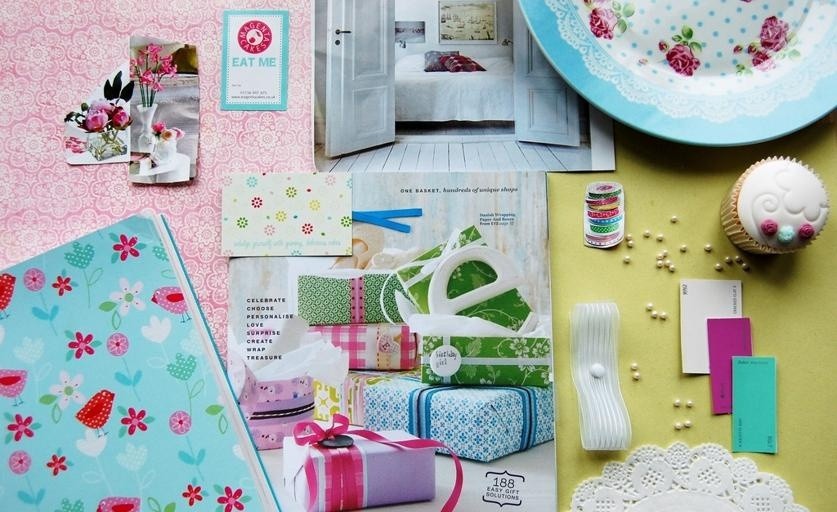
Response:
[[393, 51, 517, 130]]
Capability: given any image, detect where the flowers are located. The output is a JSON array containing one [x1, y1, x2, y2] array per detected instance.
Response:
[[130, 39, 181, 109], [151, 123, 186, 140], [64, 69, 131, 152]]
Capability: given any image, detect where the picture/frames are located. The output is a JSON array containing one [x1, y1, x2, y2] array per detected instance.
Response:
[[436, 0, 497, 45]]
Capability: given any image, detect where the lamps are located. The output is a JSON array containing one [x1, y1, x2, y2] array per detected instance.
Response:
[[395, 20, 425, 49]]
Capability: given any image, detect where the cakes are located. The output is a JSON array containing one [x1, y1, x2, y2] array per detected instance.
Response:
[[737, 159, 829, 249]]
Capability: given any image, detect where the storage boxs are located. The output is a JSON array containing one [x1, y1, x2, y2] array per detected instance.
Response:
[[237, 208, 556, 512]]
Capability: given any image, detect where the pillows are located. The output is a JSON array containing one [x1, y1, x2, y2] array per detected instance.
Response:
[[423, 48, 459, 72], [441, 55, 485, 71]]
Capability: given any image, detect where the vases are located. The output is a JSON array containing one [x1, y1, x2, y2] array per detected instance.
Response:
[[85, 130, 128, 162], [157, 138, 178, 158], [137, 102, 157, 153]]
